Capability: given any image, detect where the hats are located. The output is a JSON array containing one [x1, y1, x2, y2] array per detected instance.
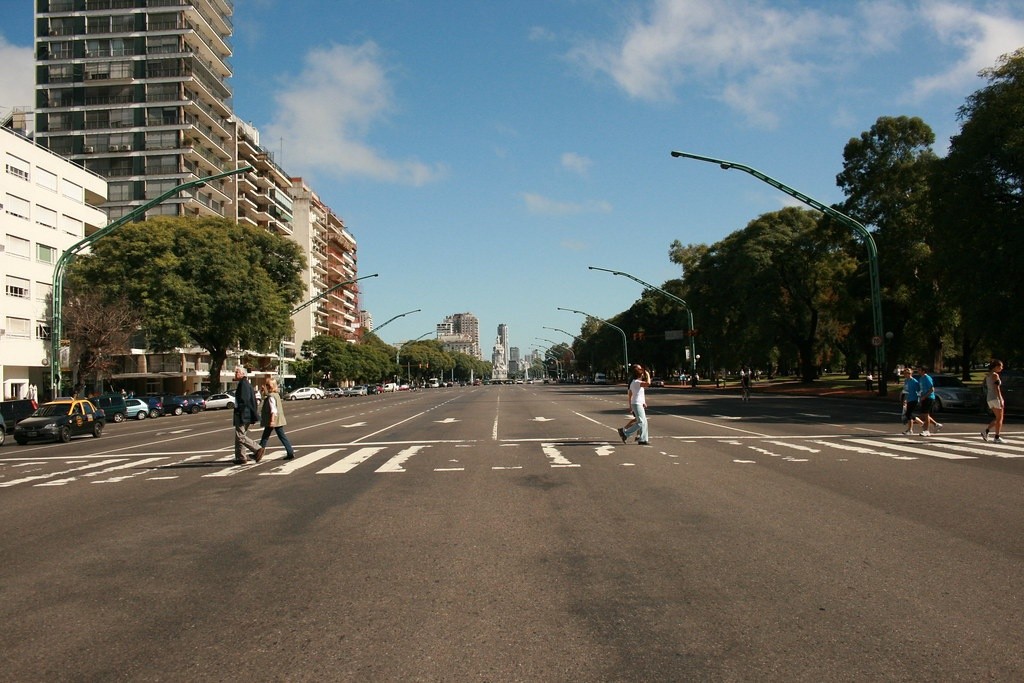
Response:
[[904, 368, 913, 373]]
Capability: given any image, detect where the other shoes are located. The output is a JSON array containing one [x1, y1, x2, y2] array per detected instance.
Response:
[[635, 437, 639, 441], [256, 448, 268, 463], [234, 460, 249, 464], [933, 423, 943, 433], [617, 428, 624, 436], [621, 434, 627, 444], [902, 429, 913, 435], [283, 454, 294, 460], [918, 430, 930, 437], [249, 454, 256, 459], [638, 442, 650, 445]]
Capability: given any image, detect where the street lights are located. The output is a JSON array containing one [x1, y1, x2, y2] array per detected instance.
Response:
[[49, 164, 258, 405], [278, 273, 379, 397], [587, 265, 698, 388], [558, 307, 629, 384], [671, 147, 890, 399], [543, 326, 596, 384], [531, 337, 575, 383], [396, 329, 435, 385]]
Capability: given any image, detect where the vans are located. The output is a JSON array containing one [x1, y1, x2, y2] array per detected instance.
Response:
[[594, 372, 606, 384], [87, 394, 128, 423]]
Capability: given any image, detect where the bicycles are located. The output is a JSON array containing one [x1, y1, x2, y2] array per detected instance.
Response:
[[740, 386, 751, 402]]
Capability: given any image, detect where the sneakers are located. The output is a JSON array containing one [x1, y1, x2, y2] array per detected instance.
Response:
[[981, 431, 989, 442], [993, 437, 1006, 443]]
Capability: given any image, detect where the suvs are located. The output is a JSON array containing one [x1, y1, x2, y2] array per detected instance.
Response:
[[0, 399, 41, 434]]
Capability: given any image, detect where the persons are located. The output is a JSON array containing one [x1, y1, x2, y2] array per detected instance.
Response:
[[866, 370, 874, 390], [748, 369, 752, 376], [980, 359, 1005, 444], [122, 388, 134, 399], [754, 369, 760, 382], [728, 370, 731, 375], [741, 373, 751, 396], [740, 369, 745, 379], [617, 364, 651, 445], [249, 379, 294, 461], [231, 365, 265, 464], [735, 371, 738, 375], [898, 369, 926, 436], [917, 364, 943, 436], [260, 384, 266, 396]]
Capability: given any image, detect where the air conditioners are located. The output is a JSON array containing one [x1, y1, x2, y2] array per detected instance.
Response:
[[83, 146, 93, 153], [121, 145, 131, 150], [108, 145, 119, 151]]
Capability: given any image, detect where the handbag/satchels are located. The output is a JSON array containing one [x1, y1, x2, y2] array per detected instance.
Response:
[[981, 378, 989, 396], [902, 404, 909, 422]]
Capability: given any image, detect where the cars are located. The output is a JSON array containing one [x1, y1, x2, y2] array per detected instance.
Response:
[[493, 379, 514, 385], [13, 397, 106, 445], [192, 390, 215, 400], [900, 375, 974, 407], [365, 382, 411, 396], [283, 387, 351, 401], [202, 394, 236, 411], [224, 388, 263, 405], [428, 378, 490, 388], [526, 379, 533, 384], [517, 379, 524, 384], [137, 396, 165, 419], [650, 377, 670, 388], [178, 394, 208, 415], [124, 398, 149, 420], [154, 394, 186, 416], [973, 376, 1024, 419], [350, 386, 368, 397]]
